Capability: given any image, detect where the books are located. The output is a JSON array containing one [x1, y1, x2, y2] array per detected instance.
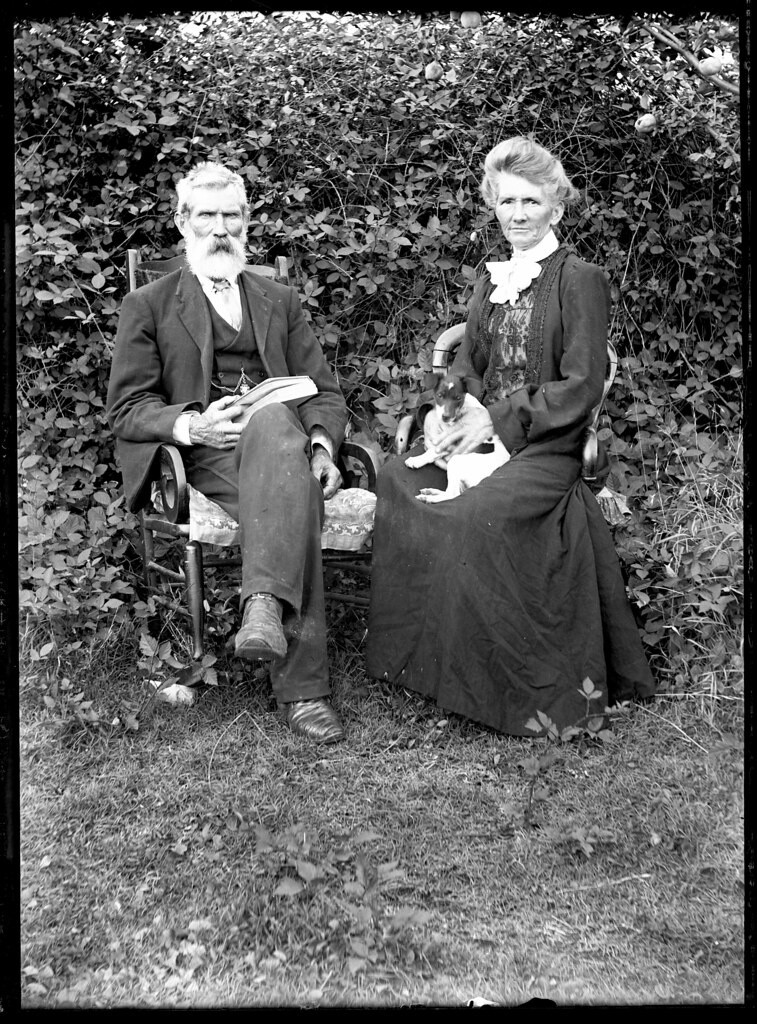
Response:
[[225, 374, 319, 424]]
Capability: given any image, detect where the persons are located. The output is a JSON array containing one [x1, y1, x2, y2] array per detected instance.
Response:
[[106, 161, 351, 744], [363, 135, 653, 737]]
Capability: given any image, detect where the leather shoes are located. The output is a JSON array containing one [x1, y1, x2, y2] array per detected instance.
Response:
[[278, 698, 345, 744], [235, 592, 288, 659]]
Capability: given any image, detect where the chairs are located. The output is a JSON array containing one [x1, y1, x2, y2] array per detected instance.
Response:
[[430, 322, 618, 494], [124, 247, 382, 659]]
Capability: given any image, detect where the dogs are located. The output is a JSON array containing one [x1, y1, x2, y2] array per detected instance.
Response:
[[404, 366, 512, 505]]
